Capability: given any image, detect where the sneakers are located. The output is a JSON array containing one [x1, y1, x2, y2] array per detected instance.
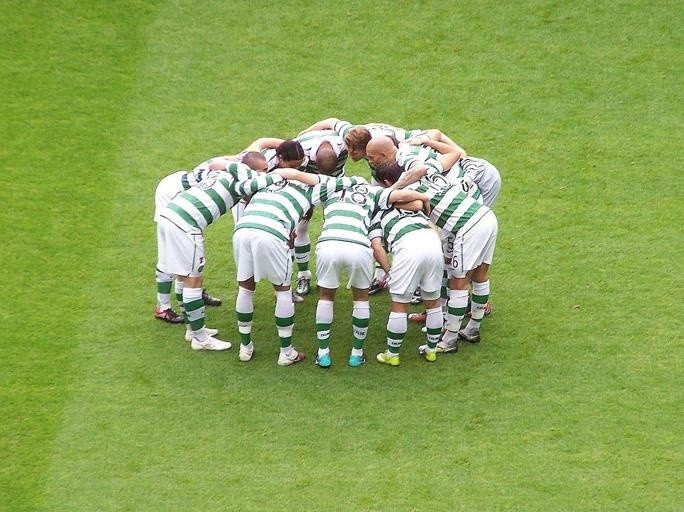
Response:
[[184, 328, 218, 341], [418, 326, 480, 361], [408, 310, 426, 321], [376, 350, 400, 366], [203, 292, 222, 306], [190, 338, 232, 351], [369, 275, 390, 295], [239, 341, 254, 361], [277, 350, 305, 367], [411, 288, 422, 304], [291, 293, 304, 303], [350, 354, 367, 367], [465, 304, 490, 316], [153, 306, 184, 323], [297, 278, 310, 295], [314, 354, 331, 367]]
